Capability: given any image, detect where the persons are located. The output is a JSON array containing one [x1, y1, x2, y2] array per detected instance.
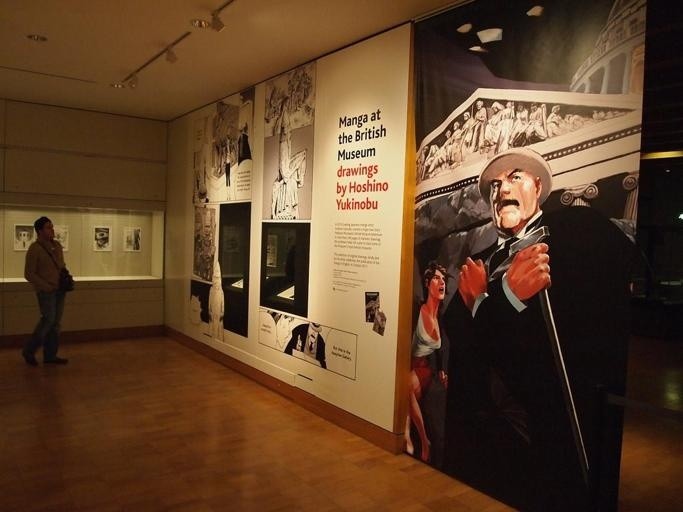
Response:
[[441, 146, 636, 511], [401, 260, 447, 461], [413, 99, 571, 185], [20, 216, 75, 367]]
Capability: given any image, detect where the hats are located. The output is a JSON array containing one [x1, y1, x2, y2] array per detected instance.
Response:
[[310, 323, 322, 332], [477, 148, 552, 205]]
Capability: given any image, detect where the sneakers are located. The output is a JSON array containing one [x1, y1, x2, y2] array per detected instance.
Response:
[[22, 351, 38, 367], [44, 358, 67, 364]]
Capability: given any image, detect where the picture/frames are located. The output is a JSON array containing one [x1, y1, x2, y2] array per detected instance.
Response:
[[92, 226, 141, 252], [13, 224, 68, 252]]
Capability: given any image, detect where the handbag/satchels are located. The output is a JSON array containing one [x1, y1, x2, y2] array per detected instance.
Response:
[[59, 268, 73, 292]]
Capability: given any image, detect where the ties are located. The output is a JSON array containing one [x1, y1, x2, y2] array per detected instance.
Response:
[[309, 342, 313, 352]]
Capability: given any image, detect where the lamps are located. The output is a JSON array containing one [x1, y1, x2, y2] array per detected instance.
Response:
[[209, 11, 225, 32]]
